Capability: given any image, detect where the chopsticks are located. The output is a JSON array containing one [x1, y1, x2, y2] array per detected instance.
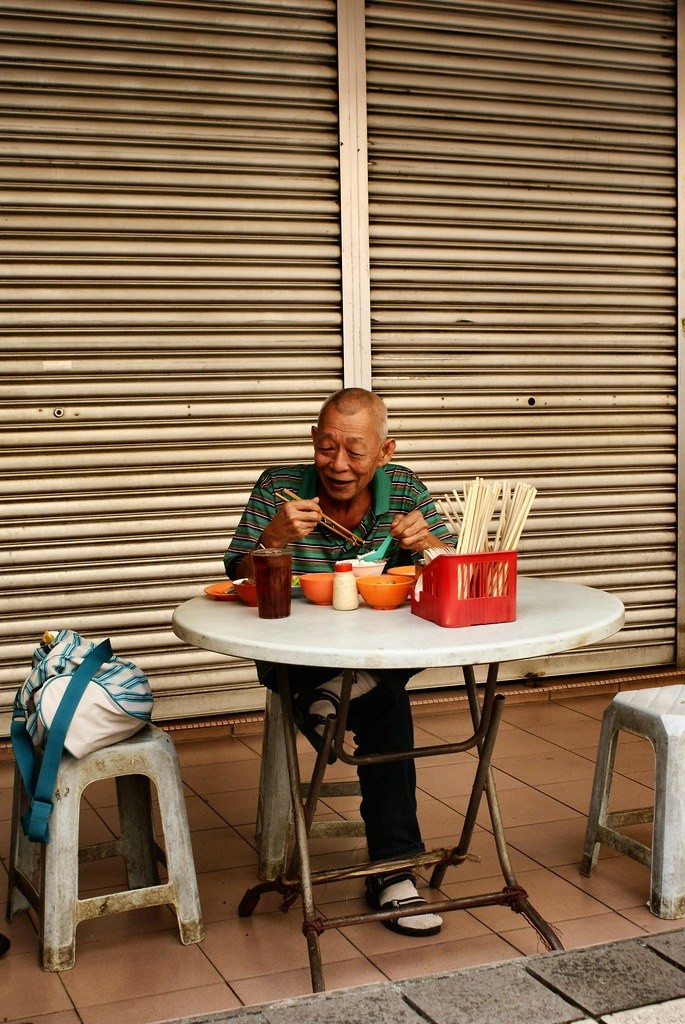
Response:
[[274, 488, 364, 547], [437, 477, 537, 624]]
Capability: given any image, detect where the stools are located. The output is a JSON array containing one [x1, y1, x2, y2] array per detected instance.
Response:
[[5, 723, 205, 973], [581, 684, 685, 920], [254, 688, 368, 882]]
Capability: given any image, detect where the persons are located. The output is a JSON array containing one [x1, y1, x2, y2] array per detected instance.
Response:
[[222, 388, 459, 937]]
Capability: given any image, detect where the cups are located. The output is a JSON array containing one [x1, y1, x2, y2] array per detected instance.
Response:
[[250, 548, 293, 619]]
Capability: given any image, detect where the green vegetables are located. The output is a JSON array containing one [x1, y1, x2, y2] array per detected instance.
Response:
[[292, 576, 300, 588]]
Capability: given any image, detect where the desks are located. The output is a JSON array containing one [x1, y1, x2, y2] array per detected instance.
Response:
[[171, 576, 625, 993]]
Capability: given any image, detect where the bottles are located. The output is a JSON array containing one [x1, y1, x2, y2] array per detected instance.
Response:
[[332, 563, 360, 610]]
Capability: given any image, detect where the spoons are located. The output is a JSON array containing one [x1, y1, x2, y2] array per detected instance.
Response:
[[360, 534, 393, 562], [424, 548, 456, 565]]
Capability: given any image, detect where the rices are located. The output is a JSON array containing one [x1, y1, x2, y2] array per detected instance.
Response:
[[347, 549, 379, 565]]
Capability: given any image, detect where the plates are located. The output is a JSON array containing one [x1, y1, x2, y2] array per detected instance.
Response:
[[203, 581, 240, 601]]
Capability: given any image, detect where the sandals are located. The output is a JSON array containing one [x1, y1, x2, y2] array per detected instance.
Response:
[[292, 688, 340, 765], [366, 868, 443, 937]]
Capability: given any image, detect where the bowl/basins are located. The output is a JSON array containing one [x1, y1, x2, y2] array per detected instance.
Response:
[[356, 575, 414, 610], [292, 586, 303, 599], [231, 578, 258, 607], [299, 573, 335, 605], [387, 565, 415, 586], [336, 559, 387, 577]]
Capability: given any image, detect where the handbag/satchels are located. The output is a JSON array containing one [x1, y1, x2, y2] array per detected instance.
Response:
[[11, 631, 154, 843]]
